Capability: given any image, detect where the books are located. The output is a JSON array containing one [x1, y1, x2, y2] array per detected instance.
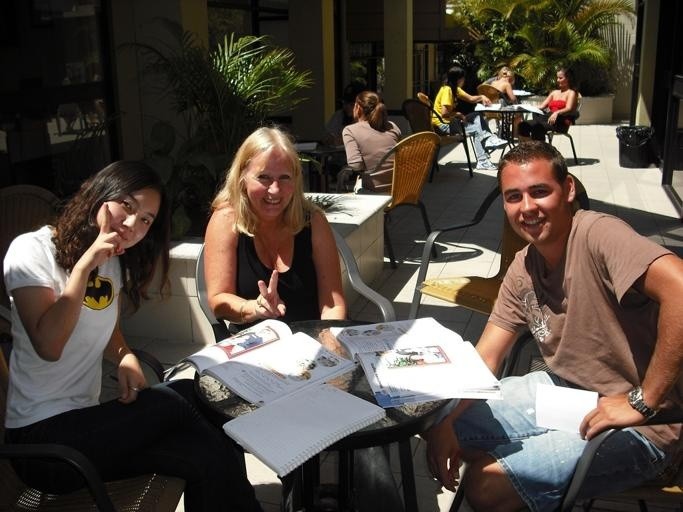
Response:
[[330, 317, 504, 409], [163, 321, 358, 406], [222, 384, 385, 477]]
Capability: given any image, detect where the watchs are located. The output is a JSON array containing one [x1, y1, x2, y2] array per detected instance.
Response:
[[627, 387, 657, 420]]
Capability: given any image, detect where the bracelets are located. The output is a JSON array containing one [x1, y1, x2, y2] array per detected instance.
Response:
[[239, 300, 249, 322]]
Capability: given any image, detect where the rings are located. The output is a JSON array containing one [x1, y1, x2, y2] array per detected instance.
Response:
[[128, 383, 143, 394]]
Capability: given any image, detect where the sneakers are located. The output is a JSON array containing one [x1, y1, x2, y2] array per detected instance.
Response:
[[484, 134, 508, 149], [476, 159, 498, 170]]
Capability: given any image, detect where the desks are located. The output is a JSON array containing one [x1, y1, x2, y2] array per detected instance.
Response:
[[499, 92, 535, 137], [301, 144, 344, 193], [474, 105, 530, 166], [193, 319, 453, 512]]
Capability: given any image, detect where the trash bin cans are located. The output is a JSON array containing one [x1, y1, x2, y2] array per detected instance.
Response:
[[615, 124, 655, 169]]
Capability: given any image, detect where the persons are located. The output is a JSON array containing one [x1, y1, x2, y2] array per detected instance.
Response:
[[342, 91, 403, 194], [204, 125, 348, 512], [325, 80, 365, 151], [431, 67, 509, 170], [3, 160, 265, 512], [518, 66, 575, 143], [488, 67, 522, 136], [483, 62, 527, 91], [427, 141, 683, 512]]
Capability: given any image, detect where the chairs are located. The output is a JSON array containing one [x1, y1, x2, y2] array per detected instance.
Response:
[[546, 93, 582, 165], [0, 306, 188, 512], [0, 185, 64, 256], [408, 172, 589, 320], [375, 116, 413, 141], [450, 329, 683, 512], [402, 99, 473, 183], [417, 91, 478, 163], [336, 132, 441, 269], [477, 85, 509, 137], [195, 222, 396, 344]]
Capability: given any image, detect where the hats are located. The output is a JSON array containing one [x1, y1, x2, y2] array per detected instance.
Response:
[[336, 85, 359, 104]]
